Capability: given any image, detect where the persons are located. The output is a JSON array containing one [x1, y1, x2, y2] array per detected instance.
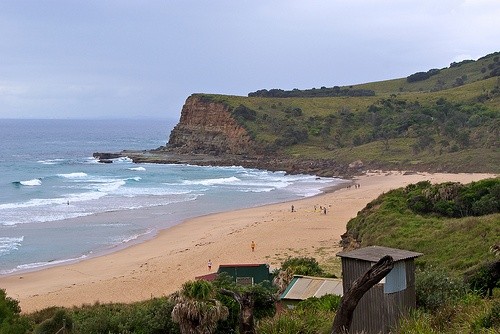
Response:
[[290, 200, 328, 216], [207, 260, 211, 271], [251, 241, 255, 252]]
